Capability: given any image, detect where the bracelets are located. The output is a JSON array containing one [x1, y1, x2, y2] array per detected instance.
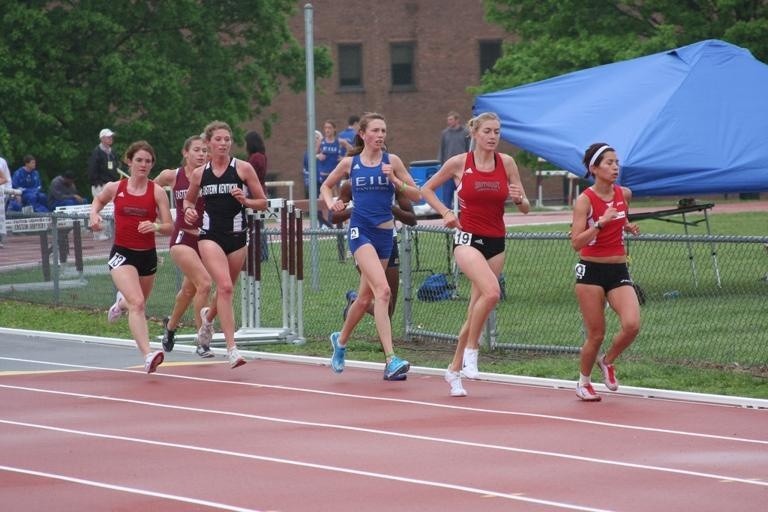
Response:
[[399, 182, 407, 192], [184, 207, 193, 212], [154, 223, 160, 232]]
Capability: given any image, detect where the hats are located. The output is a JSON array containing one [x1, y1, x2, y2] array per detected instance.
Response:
[[100, 129, 114, 137]]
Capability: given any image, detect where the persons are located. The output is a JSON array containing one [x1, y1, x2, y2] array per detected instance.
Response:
[[183, 120, 268, 369], [244, 131, 267, 262], [89, 141, 175, 375], [0, 157, 11, 247], [303, 115, 360, 199], [47, 172, 87, 210], [437, 111, 471, 209], [153, 135, 215, 359], [320, 113, 420, 380], [420, 112, 530, 397], [571, 142, 641, 401], [12, 156, 47, 208], [87, 129, 119, 198]]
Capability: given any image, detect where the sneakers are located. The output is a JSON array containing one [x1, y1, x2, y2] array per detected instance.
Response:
[[464, 348, 479, 380], [93, 233, 109, 240], [144, 351, 164, 374], [162, 317, 177, 352], [330, 332, 345, 373], [385, 358, 409, 379], [198, 307, 214, 348], [108, 291, 124, 324], [576, 383, 601, 402], [444, 364, 467, 397], [343, 291, 357, 321], [196, 341, 215, 358], [597, 354, 618, 391], [384, 374, 407, 380], [227, 347, 247, 369]]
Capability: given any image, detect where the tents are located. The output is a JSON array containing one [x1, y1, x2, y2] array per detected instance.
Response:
[[465, 38, 768, 196]]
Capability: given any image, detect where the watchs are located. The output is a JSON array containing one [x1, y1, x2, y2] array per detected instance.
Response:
[[593, 221, 602, 230], [515, 195, 523, 205]]
